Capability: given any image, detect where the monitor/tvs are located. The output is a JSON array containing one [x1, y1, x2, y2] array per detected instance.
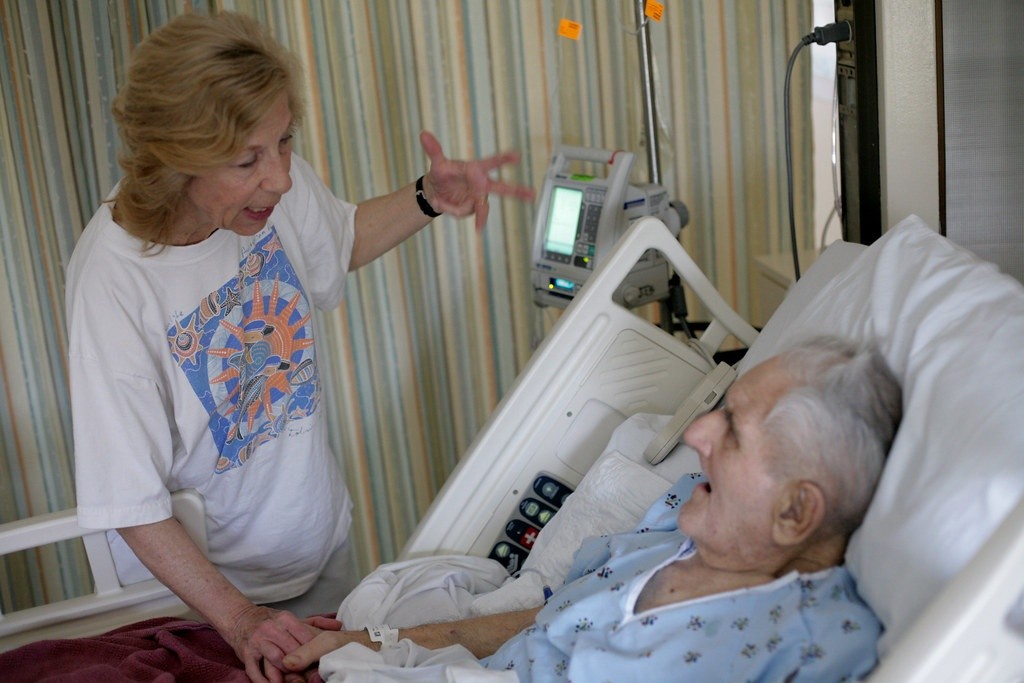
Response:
[[544, 186, 582, 265]]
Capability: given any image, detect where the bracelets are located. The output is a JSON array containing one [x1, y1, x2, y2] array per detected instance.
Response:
[[365, 623, 399, 649], [416, 174, 442, 218]]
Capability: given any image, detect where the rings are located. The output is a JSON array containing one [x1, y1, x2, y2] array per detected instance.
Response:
[[475, 197, 489, 204]]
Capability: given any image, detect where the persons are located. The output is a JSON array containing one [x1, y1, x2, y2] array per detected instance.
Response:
[[64, 12, 536, 683], [264, 331, 902, 683]]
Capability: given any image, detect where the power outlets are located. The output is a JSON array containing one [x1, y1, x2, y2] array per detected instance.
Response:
[[836, 9, 856, 52]]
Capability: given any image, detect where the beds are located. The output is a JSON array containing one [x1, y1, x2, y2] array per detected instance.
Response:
[[1, 214, 1024, 682]]
